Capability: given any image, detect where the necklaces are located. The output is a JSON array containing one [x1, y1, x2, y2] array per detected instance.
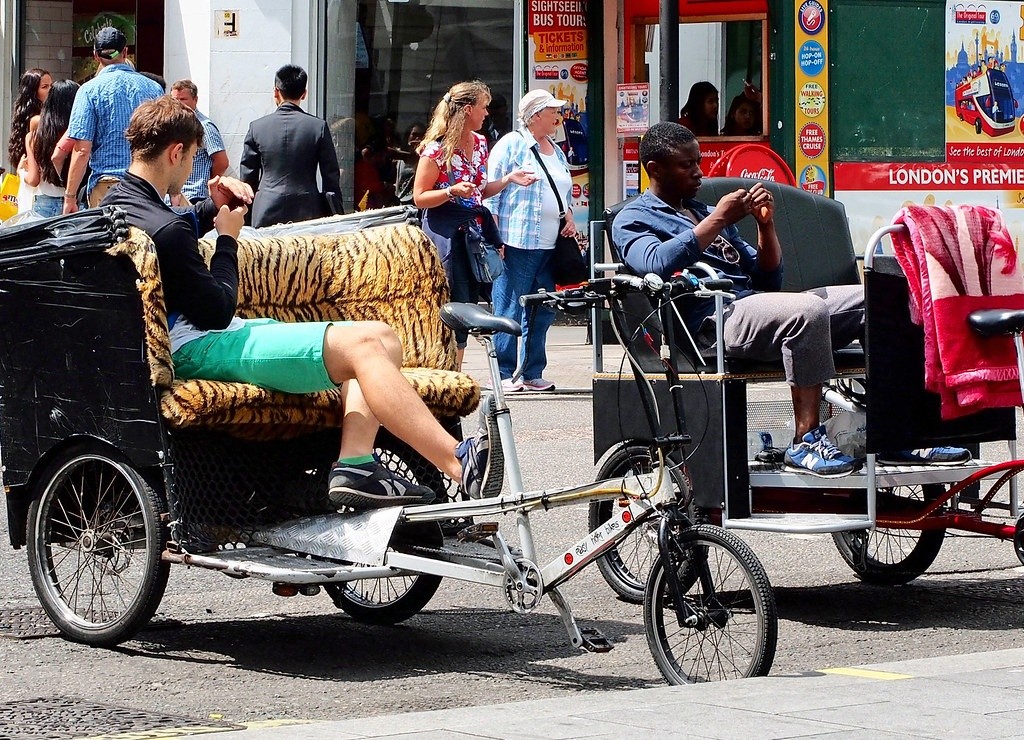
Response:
[[679, 208, 686, 213]]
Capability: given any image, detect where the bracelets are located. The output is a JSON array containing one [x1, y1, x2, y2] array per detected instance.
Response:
[[447, 187, 456, 198]]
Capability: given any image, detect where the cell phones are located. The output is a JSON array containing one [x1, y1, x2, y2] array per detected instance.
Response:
[[228, 197, 245, 212]]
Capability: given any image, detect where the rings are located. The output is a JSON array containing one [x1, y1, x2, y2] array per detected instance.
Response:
[[768, 194, 771, 200]]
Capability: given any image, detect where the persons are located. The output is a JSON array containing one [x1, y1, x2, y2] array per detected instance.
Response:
[[720, 91, 763, 136], [238, 64, 346, 229], [483, 87, 577, 392], [7, 27, 164, 217], [744, 85, 761, 103], [677, 82, 720, 136], [412, 81, 540, 372], [169, 80, 228, 205], [99, 97, 512, 511], [613, 123, 972, 478]]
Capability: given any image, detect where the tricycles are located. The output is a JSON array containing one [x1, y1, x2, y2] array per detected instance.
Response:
[[582, 176, 1023, 608], [0, 202, 780, 686]]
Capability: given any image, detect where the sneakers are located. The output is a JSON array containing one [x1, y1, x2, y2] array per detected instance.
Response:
[[454, 393, 512, 500], [522, 378, 556, 391], [875, 438, 973, 466], [780, 424, 865, 479], [484, 374, 525, 397], [327, 452, 437, 510]]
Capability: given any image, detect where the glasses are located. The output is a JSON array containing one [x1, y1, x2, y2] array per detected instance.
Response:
[[710, 235, 741, 264]]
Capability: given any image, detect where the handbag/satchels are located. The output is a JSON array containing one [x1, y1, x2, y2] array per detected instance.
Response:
[[551, 214, 589, 286], [0, 169, 34, 222], [465, 223, 508, 284]]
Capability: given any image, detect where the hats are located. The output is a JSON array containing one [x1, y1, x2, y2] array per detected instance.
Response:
[[94, 26, 127, 60], [518, 89, 568, 122]]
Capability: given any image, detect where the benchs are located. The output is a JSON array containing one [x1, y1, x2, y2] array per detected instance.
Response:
[[602, 176, 867, 381], [105, 224, 481, 439]]
